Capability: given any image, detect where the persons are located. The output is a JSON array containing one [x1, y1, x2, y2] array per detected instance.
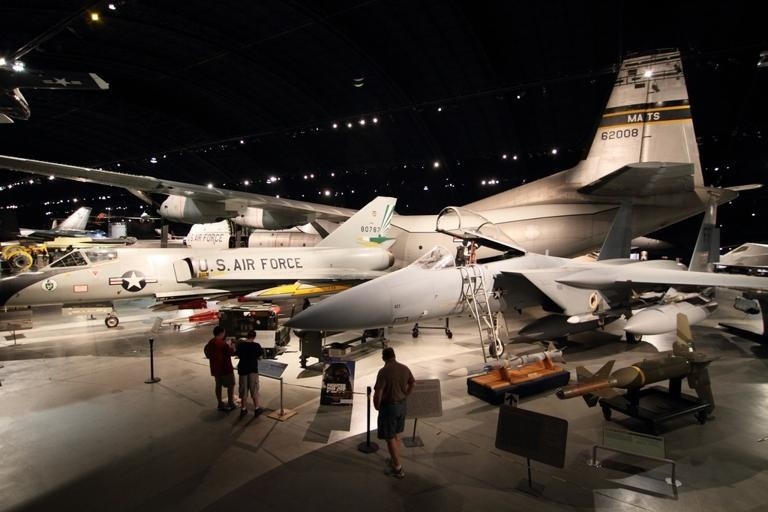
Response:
[[468, 240, 477, 264], [373, 348, 415, 480], [237, 330, 264, 418], [204, 326, 236, 408]]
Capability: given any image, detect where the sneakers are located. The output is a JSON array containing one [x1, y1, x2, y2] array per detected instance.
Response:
[[255, 407, 263, 415], [240, 408, 247, 414], [217, 402, 236, 409], [385, 460, 405, 478]]
